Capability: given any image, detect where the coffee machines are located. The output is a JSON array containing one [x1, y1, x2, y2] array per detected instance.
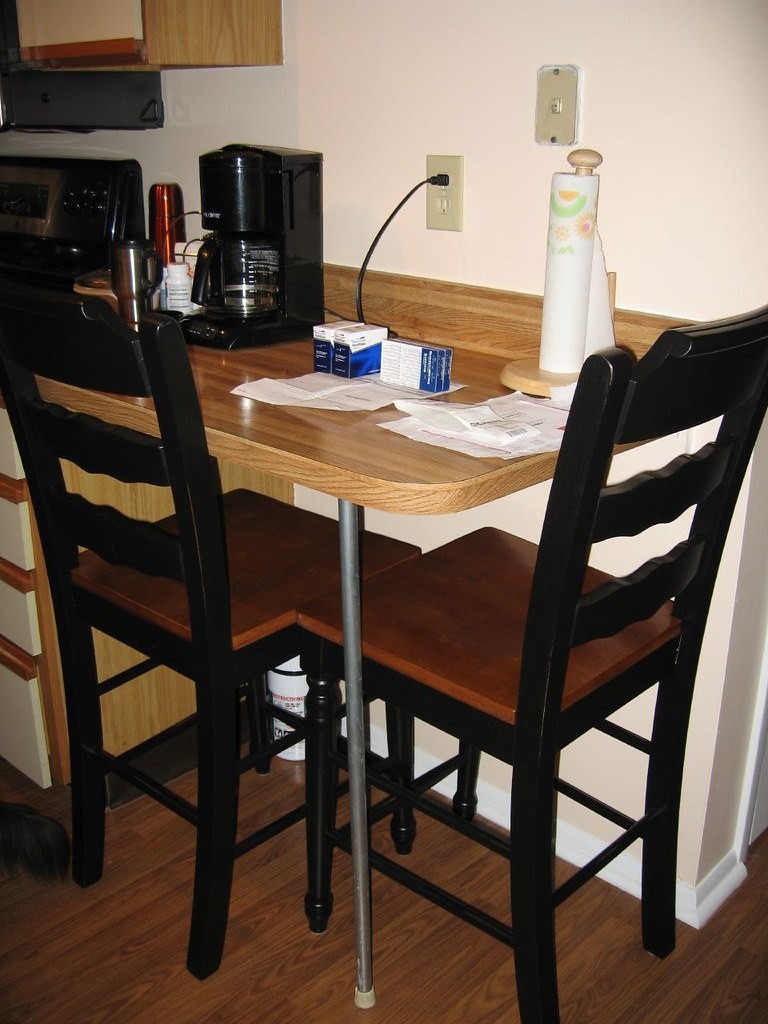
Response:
[[177, 142, 328, 348]]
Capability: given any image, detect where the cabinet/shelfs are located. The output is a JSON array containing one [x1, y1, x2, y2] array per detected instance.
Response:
[[0, 0, 282, 72], [0, 267, 708, 811], [1, 409, 295, 810]]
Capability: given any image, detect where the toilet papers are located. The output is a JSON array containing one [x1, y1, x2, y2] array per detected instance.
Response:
[[538, 169, 615, 372]]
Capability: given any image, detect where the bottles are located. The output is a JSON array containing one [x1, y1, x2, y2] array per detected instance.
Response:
[[166, 262, 193, 315]]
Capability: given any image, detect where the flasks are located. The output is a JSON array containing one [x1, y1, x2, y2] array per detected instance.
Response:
[[114, 238, 165, 323]]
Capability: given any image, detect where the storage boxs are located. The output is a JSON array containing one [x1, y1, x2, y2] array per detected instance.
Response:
[[380, 337, 452, 393], [312, 319, 388, 378]]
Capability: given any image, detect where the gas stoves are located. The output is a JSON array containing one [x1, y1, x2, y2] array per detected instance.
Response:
[[0, 154, 146, 402]]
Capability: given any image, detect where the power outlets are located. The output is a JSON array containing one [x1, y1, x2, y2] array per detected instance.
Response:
[[425, 155, 464, 232]]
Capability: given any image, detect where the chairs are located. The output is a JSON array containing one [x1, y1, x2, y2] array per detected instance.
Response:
[[1, 282, 421, 981], [294, 305, 767, 1023]]
